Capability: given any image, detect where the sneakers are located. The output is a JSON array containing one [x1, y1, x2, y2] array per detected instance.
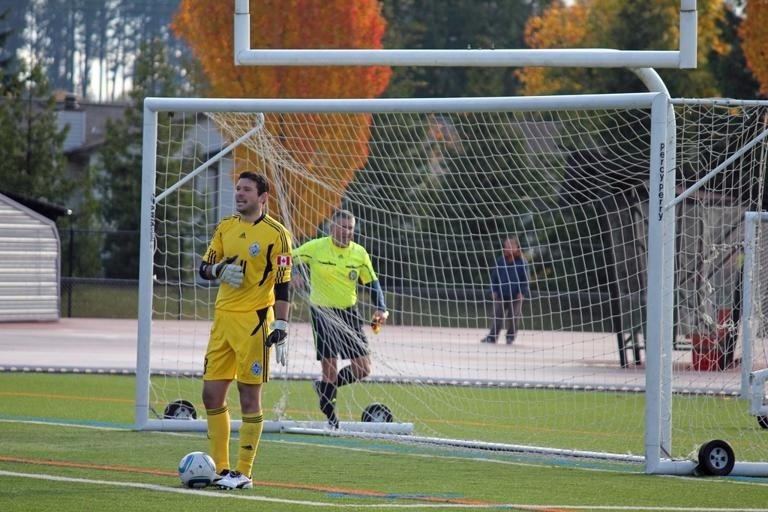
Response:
[[315, 380, 326, 413], [481, 336, 513, 345], [212, 469, 253, 491]]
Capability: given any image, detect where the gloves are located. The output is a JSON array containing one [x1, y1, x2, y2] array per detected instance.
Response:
[[265, 320, 290, 366], [212, 255, 245, 288]]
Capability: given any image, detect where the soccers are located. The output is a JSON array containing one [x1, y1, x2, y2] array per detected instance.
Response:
[[177, 452, 215, 490]]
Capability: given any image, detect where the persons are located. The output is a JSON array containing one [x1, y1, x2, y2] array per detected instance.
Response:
[[200, 169, 292, 491], [289, 210, 390, 431], [479, 235, 530, 345]]
